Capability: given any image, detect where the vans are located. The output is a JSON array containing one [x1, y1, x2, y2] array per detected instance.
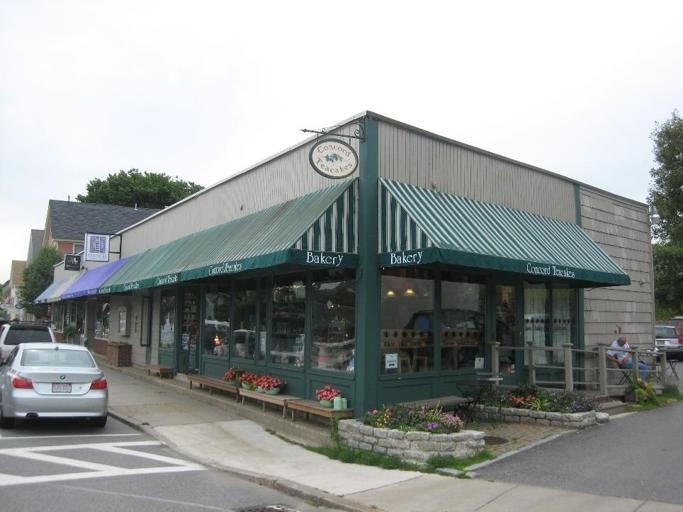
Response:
[[400, 308, 522, 370]]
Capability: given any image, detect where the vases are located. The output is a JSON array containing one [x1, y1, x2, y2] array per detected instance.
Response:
[[241, 381, 280, 395], [319, 399, 333, 407]]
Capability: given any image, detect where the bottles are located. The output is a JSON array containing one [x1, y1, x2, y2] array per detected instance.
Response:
[[333, 397, 347, 409]]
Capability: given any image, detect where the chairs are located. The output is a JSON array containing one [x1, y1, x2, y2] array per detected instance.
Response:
[[615, 357, 634, 385], [665, 358, 681, 382]]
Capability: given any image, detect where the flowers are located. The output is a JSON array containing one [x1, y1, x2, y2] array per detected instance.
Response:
[[240, 372, 281, 387], [315, 385, 341, 400]]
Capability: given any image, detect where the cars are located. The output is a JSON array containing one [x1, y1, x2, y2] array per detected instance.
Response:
[[1, 316, 108, 428], [213, 329, 256, 361], [654, 325, 682, 362]]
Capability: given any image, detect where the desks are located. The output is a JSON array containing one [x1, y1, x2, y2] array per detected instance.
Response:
[[443, 379, 497, 427]]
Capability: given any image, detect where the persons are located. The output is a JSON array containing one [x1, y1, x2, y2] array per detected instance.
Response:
[[606, 335, 650, 387]]
